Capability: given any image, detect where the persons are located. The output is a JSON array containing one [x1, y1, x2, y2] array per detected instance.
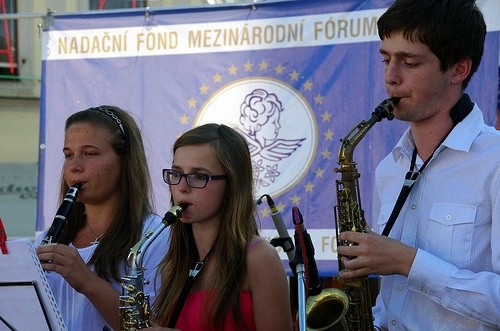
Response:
[[334, 0, 500, 331], [151, 123, 294, 331], [33, 106, 175, 331]]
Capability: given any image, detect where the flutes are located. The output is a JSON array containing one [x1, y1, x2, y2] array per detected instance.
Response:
[[36, 182, 83, 273]]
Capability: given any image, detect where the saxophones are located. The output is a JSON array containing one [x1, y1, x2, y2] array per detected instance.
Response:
[[116, 202, 189, 331], [294, 95, 403, 331]]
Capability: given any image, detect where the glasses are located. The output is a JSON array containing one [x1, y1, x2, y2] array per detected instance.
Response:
[[163, 169, 226, 188]]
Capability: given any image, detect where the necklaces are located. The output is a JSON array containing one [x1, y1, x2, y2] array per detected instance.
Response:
[[86, 219, 102, 245]]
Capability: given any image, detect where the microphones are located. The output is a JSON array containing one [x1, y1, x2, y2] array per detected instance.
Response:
[[269, 199, 297, 277]]
